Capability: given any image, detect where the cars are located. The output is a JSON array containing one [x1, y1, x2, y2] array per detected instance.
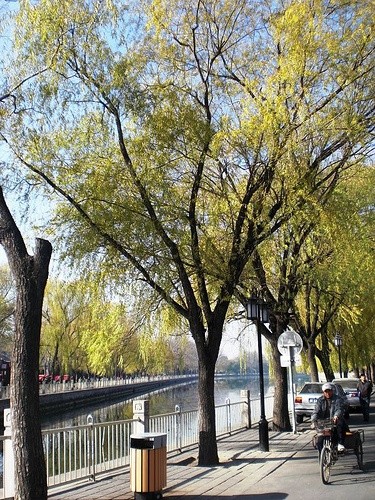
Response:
[[294, 381, 332, 424], [332, 377, 370, 418]]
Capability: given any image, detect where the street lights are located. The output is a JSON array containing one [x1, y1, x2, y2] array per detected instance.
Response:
[[247, 294, 272, 451], [335, 330, 342, 378]]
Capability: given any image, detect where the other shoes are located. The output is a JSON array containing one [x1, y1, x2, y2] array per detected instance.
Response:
[[338, 444, 344, 451]]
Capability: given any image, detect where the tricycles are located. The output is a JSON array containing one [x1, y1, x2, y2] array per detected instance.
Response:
[[312, 417, 365, 485]]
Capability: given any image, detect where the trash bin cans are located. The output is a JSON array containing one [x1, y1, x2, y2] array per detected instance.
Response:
[[130, 432, 167, 500]]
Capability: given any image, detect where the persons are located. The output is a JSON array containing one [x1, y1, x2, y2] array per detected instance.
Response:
[[356, 374, 372, 422], [310, 384, 345, 453]]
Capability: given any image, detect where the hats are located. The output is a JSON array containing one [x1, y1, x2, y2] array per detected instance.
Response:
[[322, 383, 331, 392]]
